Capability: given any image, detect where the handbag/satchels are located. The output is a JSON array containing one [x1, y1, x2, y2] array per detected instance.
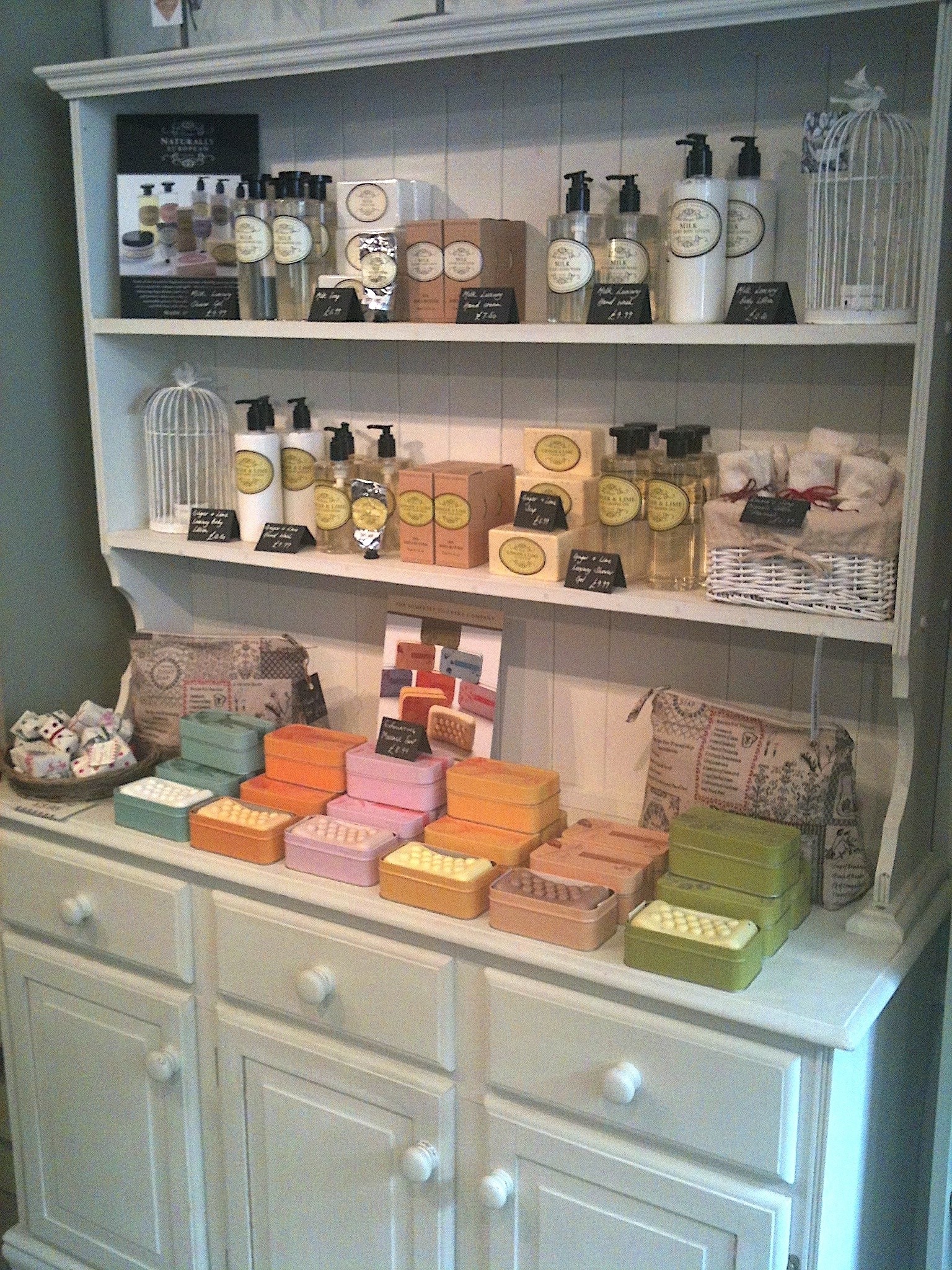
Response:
[[624, 685, 873, 911], [126, 629, 309, 764]]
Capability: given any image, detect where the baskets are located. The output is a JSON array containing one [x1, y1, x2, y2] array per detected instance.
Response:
[[702, 495, 895, 621], [0, 734, 159, 801]]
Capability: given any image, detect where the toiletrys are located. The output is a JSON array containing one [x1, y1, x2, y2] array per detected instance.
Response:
[[665, 129, 776, 325], [139, 174, 233, 259], [595, 417, 723, 594], [233, 391, 413, 555], [233, 170, 334, 321], [546, 168, 664, 322]]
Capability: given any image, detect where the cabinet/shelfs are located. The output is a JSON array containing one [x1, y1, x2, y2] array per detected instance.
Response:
[[478, 964, 802, 1270], [209, 887, 458, 1270], [35, 0, 952, 931], [0, 831, 206, 1269]]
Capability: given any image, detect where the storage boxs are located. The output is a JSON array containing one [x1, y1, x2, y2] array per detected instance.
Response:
[[406, 217, 526, 322], [398, 459, 515, 568]]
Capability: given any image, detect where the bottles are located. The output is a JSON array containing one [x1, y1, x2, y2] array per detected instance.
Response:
[[137, 181, 178, 246], [231, 172, 336, 322], [599, 422, 721, 591]]
